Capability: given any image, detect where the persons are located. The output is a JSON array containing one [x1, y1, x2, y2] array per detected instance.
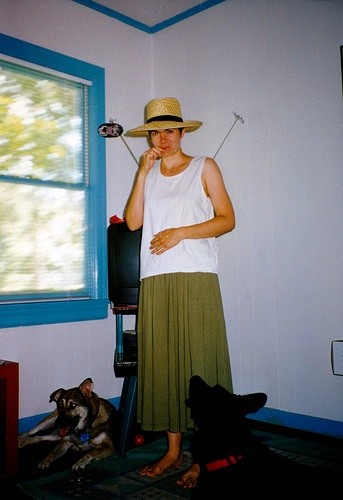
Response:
[[126, 95, 235, 489]]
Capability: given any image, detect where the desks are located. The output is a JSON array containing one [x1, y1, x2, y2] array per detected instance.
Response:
[[111, 306, 143, 459]]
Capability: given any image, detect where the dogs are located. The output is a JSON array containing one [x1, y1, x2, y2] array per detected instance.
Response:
[[184, 375, 267, 500], [18, 377, 119, 472]]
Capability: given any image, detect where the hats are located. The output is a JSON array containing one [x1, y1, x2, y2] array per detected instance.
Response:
[[125, 96, 203, 138]]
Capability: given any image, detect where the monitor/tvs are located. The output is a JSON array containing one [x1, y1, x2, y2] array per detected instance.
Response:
[[107, 222, 142, 305]]
